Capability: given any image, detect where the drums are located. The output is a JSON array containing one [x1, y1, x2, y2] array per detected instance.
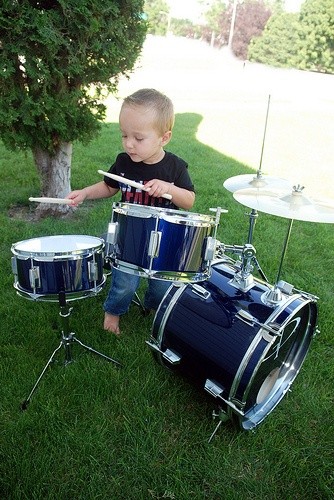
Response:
[[10, 234, 106, 303], [105, 203, 218, 283], [147, 257, 319, 434]]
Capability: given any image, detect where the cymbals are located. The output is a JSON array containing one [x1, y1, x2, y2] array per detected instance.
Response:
[[234, 186, 333, 224], [223, 173, 291, 193]]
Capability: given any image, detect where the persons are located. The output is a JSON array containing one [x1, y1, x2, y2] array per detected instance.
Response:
[[66, 89, 194, 335]]
